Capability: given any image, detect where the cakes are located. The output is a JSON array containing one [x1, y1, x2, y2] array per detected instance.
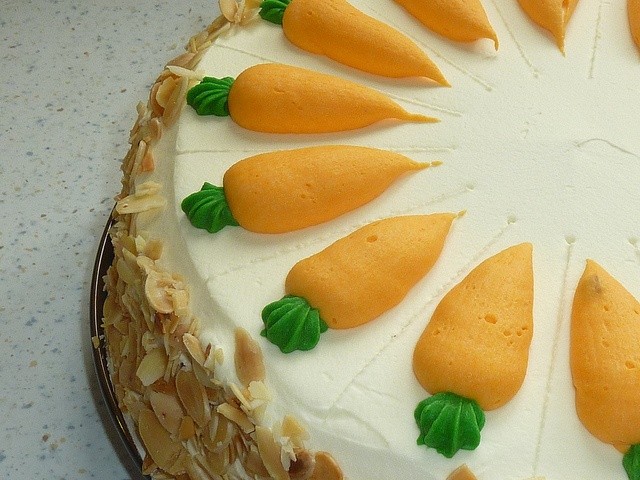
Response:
[[93, 0, 640, 480]]
